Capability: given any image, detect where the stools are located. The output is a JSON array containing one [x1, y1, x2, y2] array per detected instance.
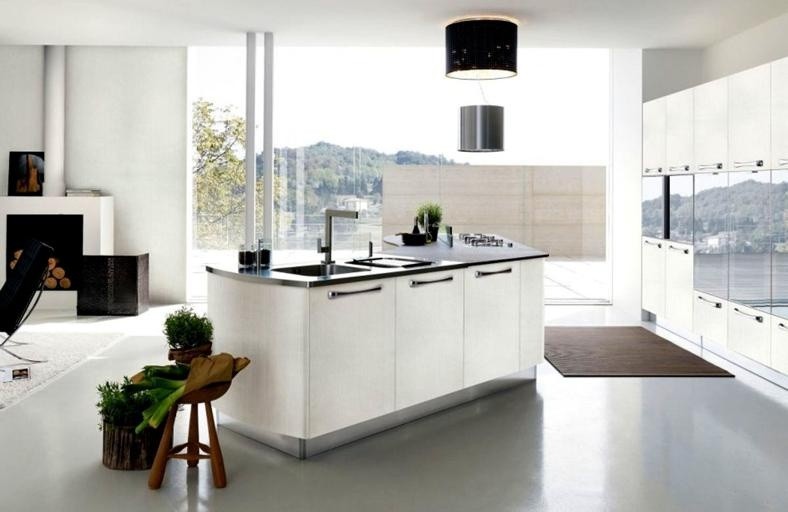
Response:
[[75, 251, 152, 316], [124, 364, 237, 492]]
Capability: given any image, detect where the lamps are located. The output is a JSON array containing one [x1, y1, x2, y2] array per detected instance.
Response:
[[442, 15, 520, 82], [456, 103, 505, 155]]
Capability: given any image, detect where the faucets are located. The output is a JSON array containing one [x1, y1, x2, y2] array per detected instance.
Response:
[[318, 207, 358, 264]]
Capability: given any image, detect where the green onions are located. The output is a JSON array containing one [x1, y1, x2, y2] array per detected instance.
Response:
[[135, 376, 187, 435]]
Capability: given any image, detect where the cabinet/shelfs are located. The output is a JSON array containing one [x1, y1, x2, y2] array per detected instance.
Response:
[[638, 59, 788, 396], [205, 264, 398, 464], [393, 263, 468, 429], [461, 254, 548, 392]]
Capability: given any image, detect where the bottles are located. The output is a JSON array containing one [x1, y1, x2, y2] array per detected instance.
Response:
[[412, 217, 420, 233]]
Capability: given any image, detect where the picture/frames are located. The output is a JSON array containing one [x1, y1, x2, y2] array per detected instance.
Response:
[[7, 150, 46, 197]]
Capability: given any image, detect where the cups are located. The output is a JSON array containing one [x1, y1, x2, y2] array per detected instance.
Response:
[[238, 244, 254, 269], [257, 239, 272, 269]]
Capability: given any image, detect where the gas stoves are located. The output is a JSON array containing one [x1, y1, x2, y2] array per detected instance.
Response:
[[453, 232, 514, 248]]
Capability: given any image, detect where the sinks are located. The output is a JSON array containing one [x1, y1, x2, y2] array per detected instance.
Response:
[[273, 263, 370, 277]]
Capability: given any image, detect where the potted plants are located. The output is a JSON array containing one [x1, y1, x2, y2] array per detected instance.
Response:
[[414, 199, 446, 244], [161, 304, 216, 366], [92, 372, 175, 472]]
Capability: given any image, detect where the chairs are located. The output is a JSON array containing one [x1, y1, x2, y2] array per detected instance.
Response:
[[0, 237, 59, 366]]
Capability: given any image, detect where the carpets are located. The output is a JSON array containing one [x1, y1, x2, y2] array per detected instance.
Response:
[[0, 307, 164, 416], [540, 321, 737, 382]]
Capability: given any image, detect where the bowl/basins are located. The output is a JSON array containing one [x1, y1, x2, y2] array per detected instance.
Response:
[[402, 233, 426, 245]]
